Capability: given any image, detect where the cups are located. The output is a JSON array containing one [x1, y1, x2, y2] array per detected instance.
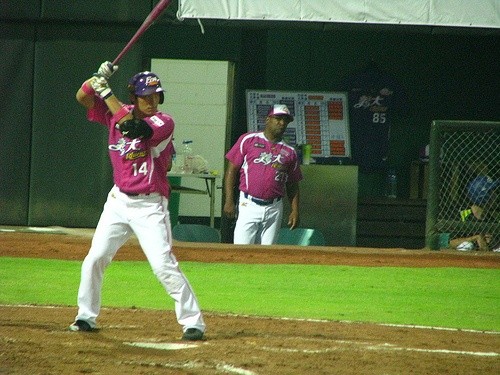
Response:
[[301, 144, 312, 165]]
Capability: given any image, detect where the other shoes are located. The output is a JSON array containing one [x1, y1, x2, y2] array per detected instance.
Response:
[[68, 320, 100, 332], [181, 328, 204, 341]]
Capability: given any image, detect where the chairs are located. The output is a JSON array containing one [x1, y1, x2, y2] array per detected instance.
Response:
[[275, 227, 327, 246], [172, 223, 223, 243]]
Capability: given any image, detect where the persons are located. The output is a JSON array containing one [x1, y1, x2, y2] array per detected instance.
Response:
[[438, 174, 500, 251], [337, 50, 407, 199], [69, 60, 207, 340], [223, 104, 304, 246]]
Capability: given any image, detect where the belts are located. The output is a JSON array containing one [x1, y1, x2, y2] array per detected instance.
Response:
[[244, 193, 281, 205], [119, 188, 150, 197]]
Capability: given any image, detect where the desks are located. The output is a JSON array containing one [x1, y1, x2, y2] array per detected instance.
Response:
[[166, 172, 221, 228], [282, 164, 359, 246], [413, 157, 470, 203]]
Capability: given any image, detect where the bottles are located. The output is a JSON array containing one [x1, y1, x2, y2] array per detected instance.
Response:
[[169, 138, 176, 169], [181, 140, 193, 174]]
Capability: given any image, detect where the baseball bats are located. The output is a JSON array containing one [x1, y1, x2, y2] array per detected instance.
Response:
[[111, 0, 171, 66]]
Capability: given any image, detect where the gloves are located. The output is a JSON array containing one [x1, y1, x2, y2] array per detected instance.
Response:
[[97, 61, 120, 79], [90, 77, 112, 99]]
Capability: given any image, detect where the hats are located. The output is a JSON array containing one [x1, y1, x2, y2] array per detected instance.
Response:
[[467, 176, 500, 204], [266, 103, 295, 122], [128, 70, 165, 104]]
